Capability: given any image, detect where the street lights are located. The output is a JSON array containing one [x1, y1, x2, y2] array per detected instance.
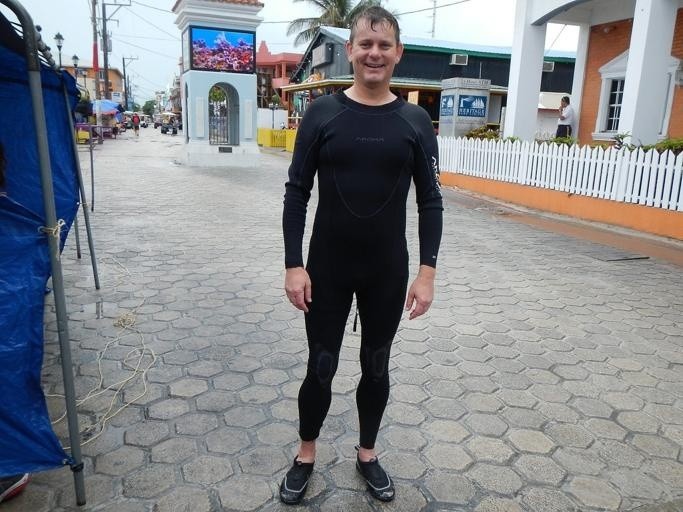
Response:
[[53, 32, 63, 69], [71, 52, 79, 83], [81, 67, 89, 124], [268, 101, 278, 130]]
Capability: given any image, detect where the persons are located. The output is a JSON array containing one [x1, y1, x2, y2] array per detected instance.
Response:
[[131, 113, 140, 136], [555, 96, 573, 138], [278, 5, 444, 504]]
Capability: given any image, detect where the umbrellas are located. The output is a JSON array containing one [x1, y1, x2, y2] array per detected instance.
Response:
[[92, 104, 119, 125]]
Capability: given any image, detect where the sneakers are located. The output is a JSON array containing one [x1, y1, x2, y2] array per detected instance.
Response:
[[279, 455, 315, 505], [354, 445, 396, 502]]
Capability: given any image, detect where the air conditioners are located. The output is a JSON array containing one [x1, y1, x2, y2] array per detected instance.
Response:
[[450, 53, 468, 66], [541, 61, 555, 73]]
